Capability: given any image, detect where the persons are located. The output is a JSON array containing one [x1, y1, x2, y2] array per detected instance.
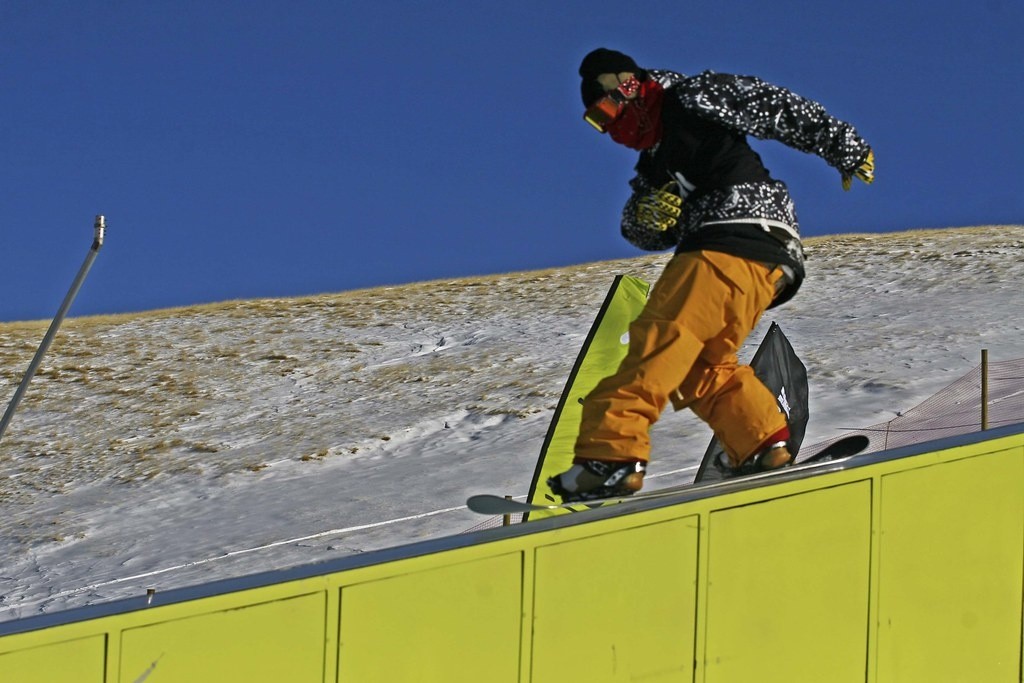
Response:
[[550, 48, 876, 501]]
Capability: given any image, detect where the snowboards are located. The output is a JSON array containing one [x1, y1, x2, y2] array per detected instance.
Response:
[[465, 435, 872, 517]]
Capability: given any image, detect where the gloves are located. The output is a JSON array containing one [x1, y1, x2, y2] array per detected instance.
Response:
[[629, 185, 682, 233], [842, 148, 875, 191]]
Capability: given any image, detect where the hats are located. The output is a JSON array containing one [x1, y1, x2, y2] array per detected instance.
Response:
[[579, 48, 643, 108]]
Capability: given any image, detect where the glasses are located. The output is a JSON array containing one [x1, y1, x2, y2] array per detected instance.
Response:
[[583, 94, 626, 133]]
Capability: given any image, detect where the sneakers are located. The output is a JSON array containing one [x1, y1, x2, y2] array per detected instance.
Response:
[[713, 441, 792, 478], [546, 457, 647, 502]]
